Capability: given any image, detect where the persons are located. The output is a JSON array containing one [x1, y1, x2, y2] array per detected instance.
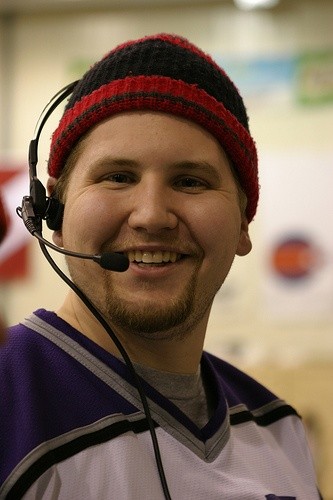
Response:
[[0, 34, 324, 500]]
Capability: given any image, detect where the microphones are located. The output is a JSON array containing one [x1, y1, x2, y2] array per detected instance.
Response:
[[22, 195, 130, 273]]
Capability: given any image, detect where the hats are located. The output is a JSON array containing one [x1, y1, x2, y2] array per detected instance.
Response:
[[47, 32, 259, 224]]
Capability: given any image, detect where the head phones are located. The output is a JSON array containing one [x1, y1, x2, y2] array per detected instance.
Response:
[[29, 79, 82, 237]]
